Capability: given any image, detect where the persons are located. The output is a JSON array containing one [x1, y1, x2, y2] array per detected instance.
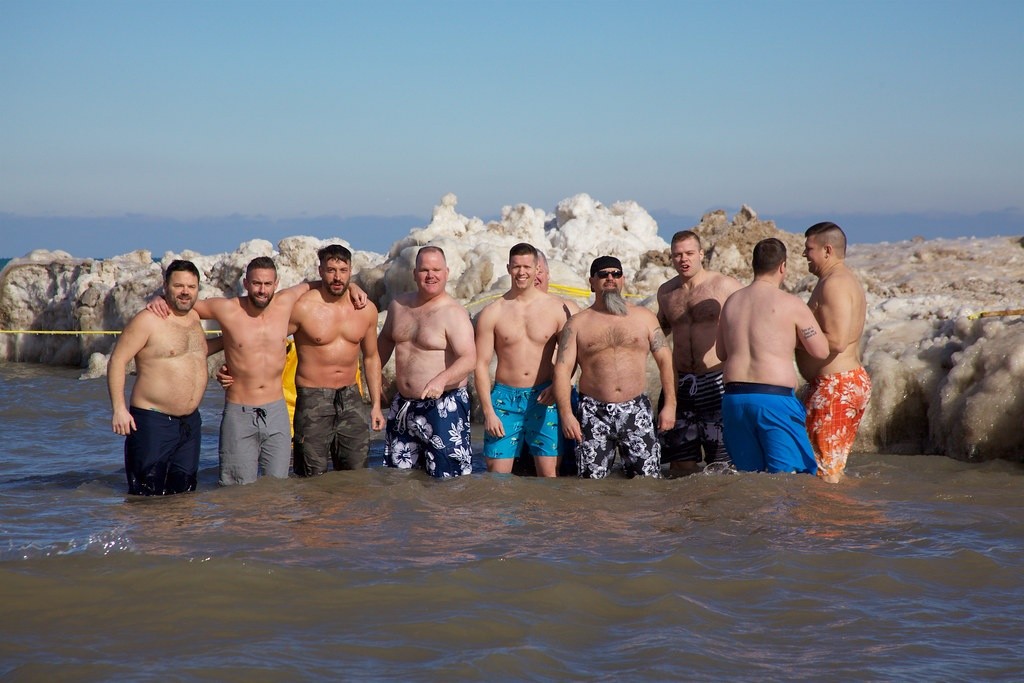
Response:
[[655, 231, 745, 477], [714, 238, 830, 476], [470, 243, 579, 479], [551, 255, 678, 479], [792, 221, 875, 486], [104, 258, 225, 498], [215, 243, 386, 482], [376, 247, 478, 484], [142, 255, 369, 488], [528, 247, 583, 318]]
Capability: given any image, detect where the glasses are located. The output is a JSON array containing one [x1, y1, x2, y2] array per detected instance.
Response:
[[597, 269, 623, 279]]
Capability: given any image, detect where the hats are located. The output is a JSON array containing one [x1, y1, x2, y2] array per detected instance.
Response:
[[591, 256, 622, 291]]
